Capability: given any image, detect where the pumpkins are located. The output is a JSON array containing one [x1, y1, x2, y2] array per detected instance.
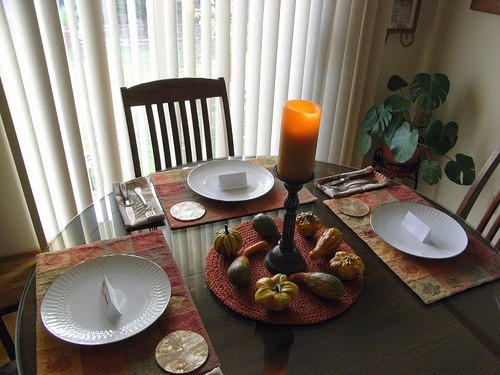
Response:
[[213, 211, 364, 312]]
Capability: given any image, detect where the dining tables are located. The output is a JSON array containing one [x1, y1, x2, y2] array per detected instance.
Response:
[[11, 154, 500, 375]]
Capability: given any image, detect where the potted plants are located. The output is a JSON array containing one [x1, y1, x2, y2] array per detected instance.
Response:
[[358, 71, 477, 186]]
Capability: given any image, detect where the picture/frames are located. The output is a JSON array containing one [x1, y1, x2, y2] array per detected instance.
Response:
[[387, 0, 422, 33]]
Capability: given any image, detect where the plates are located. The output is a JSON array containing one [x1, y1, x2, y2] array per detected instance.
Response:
[[187, 159, 274, 201], [40, 254, 171, 345], [370, 202, 468, 259]]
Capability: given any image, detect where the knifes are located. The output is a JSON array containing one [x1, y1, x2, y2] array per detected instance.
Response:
[[328, 170, 374, 186], [120, 182, 135, 225]]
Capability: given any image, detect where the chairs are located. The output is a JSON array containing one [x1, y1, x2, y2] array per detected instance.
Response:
[[119, 76, 243, 177], [455, 144, 500, 251]]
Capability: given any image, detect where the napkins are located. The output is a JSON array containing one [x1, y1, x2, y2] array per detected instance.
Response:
[[315, 166, 392, 198], [111, 175, 166, 228]]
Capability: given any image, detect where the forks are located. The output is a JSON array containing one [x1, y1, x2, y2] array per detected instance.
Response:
[[332, 180, 378, 191], [134, 186, 156, 218]]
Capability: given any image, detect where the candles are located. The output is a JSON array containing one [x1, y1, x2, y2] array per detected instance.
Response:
[[277, 95, 325, 178]]
